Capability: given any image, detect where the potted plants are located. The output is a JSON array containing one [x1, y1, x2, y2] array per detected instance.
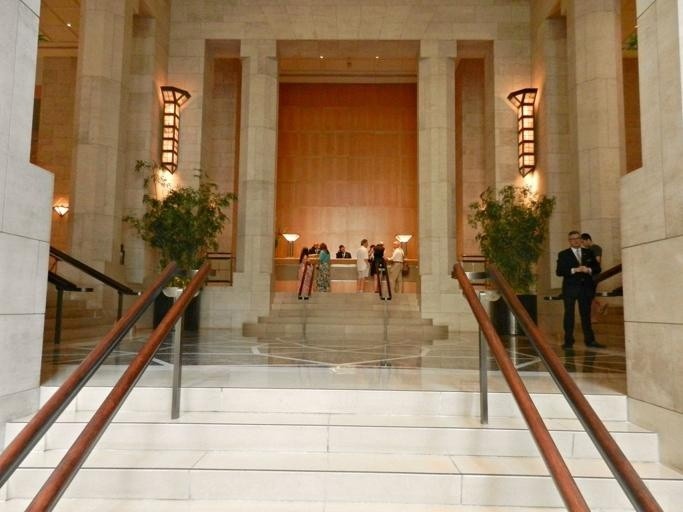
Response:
[[466, 185, 557, 335], [119, 156, 238, 336]]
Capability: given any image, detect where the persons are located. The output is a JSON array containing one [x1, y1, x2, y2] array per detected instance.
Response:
[[356, 239, 370, 293], [308, 240, 321, 254], [580, 232, 603, 298], [371, 240, 388, 293], [315, 240, 331, 292], [297, 246, 314, 300], [369, 245, 375, 258], [336, 245, 351, 258], [382, 241, 405, 293], [555, 230, 607, 349]]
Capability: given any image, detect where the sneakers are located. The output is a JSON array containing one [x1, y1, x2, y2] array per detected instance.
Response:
[[563, 341, 573, 348], [586, 340, 606, 349]]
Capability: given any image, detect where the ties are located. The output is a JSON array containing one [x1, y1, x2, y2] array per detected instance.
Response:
[[576, 249, 582, 265]]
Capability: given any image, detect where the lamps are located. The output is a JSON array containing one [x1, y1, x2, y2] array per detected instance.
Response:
[[282, 234, 300, 257], [506, 88, 538, 177], [53, 206, 68, 216], [160, 85, 192, 175], [394, 234, 413, 257]]
